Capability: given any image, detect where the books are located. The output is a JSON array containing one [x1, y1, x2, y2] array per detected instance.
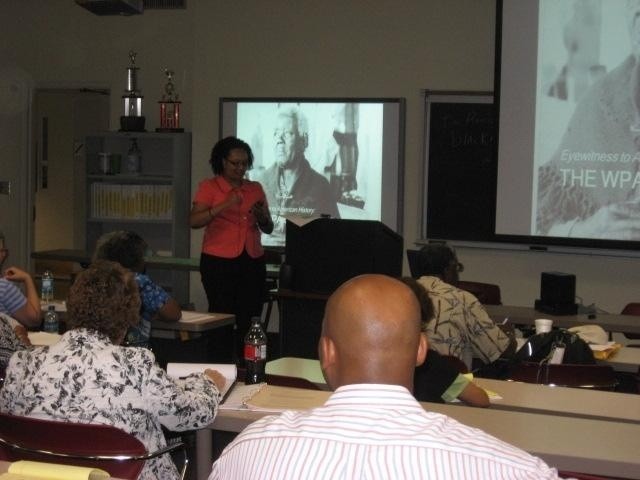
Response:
[[89, 180, 175, 221]]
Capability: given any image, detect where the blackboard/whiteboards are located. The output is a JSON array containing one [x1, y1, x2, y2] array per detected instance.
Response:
[[422, 89, 640, 258]]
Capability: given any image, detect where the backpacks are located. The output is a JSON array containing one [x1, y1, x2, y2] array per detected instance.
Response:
[[497, 329, 596, 382]]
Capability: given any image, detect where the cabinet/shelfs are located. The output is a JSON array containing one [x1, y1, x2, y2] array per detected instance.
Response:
[[80, 132, 193, 317]]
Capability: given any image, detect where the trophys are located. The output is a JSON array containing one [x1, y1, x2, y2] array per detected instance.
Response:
[[153, 63, 185, 132], [118, 47, 149, 132]]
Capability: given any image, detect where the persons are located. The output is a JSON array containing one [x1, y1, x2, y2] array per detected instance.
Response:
[[2, 259, 227, 480], [0, 233, 43, 328], [189, 135, 275, 356], [400, 276, 489, 408], [538, 0, 638, 241], [416, 240, 518, 374], [92, 229, 182, 349], [257, 105, 342, 241], [207, 271, 580, 480], [544, 15, 605, 103], [0, 315, 34, 374]]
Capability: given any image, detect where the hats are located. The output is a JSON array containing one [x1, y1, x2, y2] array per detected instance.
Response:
[[567, 324, 616, 352]]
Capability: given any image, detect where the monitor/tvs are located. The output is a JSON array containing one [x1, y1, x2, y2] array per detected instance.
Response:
[[406, 249, 426, 278]]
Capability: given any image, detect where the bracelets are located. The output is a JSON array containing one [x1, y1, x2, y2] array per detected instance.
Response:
[[505, 331, 515, 338], [259, 218, 269, 228], [18, 334, 28, 340], [209, 208, 217, 219]]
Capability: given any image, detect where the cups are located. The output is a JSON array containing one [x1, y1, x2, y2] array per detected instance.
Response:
[[534, 318, 555, 335], [98, 151, 122, 176]]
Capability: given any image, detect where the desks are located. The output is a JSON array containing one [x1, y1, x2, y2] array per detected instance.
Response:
[[0, 273, 640, 479]]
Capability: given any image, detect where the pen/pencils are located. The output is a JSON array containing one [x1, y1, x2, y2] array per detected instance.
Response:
[[428, 241, 447, 244], [530, 246, 548, 250], [179, 377, 187, 379]]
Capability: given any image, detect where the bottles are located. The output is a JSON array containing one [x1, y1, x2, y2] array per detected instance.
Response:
[[40, 267, 54, 307], [43, 306, 59, 334], [243, 315, 268, 385]]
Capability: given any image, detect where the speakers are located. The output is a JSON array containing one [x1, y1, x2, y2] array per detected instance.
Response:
[[534, 272, 578, 316]]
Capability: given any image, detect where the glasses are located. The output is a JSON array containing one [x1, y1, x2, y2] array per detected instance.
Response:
[[225, 157, 249, 168]]
